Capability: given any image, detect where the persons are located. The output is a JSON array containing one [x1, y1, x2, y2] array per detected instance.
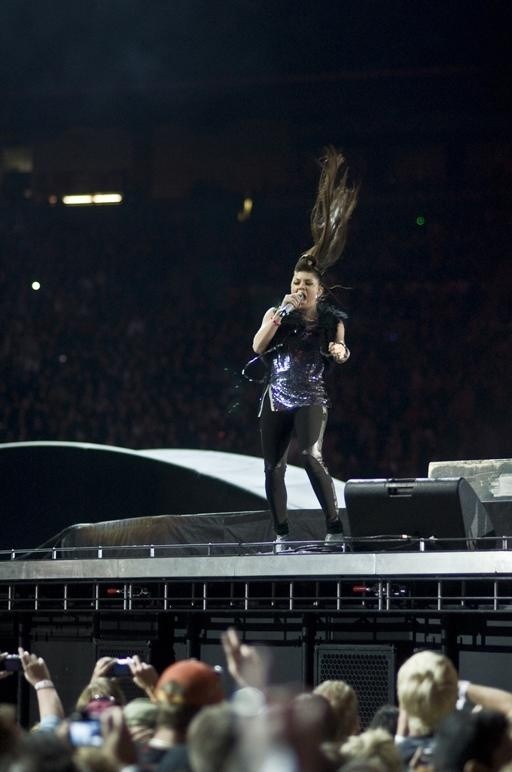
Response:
[[251, 141, 363, 553]]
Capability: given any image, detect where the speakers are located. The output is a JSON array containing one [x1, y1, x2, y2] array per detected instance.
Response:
[[343, 479, 496, 551]]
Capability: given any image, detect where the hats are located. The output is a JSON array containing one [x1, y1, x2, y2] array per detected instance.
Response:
[[151, 656, 223, 710]]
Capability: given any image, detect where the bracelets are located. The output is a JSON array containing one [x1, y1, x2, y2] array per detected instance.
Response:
[[270, 317, 282, 328], [336, 341, 347, 349]]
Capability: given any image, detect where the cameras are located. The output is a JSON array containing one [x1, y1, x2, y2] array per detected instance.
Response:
[[69, 719, 105, 748], [110, 660, 133, 678], [3, 656, 22, 672]]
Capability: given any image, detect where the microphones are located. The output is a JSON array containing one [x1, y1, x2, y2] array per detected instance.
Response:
[[279, 293, 303, 316]]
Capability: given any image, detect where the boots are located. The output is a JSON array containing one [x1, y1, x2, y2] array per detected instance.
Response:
[[273, 524, 291, 554], [322, 519, 345, 548]]
